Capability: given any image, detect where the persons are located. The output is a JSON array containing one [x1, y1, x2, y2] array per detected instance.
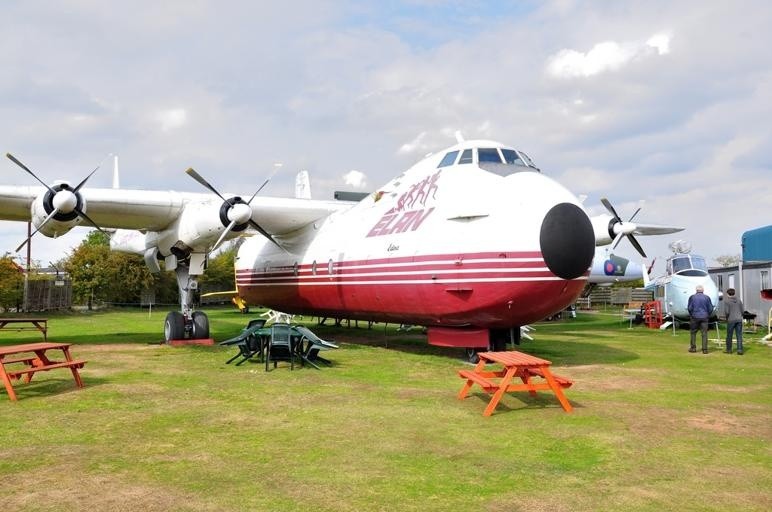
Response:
[[687, 286, 713, 354], [724, 288, 745, 355]]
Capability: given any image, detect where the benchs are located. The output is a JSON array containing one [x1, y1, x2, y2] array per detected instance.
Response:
[[457, 369, 575, 393], [0, 326, 50, 332], [0, 357, 87, 381]]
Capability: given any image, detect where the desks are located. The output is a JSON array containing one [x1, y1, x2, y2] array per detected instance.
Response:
[[0, 340, 84, 401], [0, 318, 48, 344], [458, 351, 572, 416]]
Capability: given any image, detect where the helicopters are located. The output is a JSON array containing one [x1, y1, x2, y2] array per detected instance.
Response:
[[641, 256, 724, 330]]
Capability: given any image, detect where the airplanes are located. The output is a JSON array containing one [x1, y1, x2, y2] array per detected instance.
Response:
[[0, 138, 685, 364]]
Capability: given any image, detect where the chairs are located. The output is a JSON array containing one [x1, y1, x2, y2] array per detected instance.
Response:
[[219, 309, 339, 372]]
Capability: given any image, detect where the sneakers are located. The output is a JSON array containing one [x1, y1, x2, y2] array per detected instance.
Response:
[[688, 348, 696, 352], [703, 350, 708, 354], [722, 349, 732, 354], [737, 351, 743, 355]]
